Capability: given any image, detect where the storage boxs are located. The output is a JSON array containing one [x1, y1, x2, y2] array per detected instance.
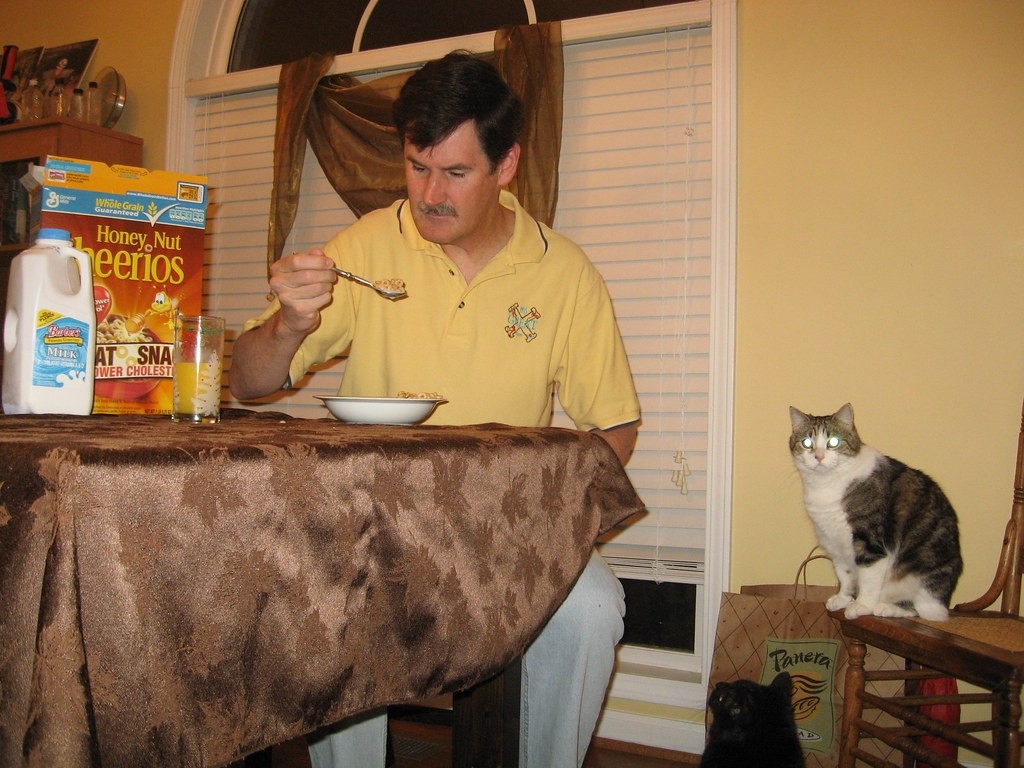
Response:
[[19, 154, 209, 415]]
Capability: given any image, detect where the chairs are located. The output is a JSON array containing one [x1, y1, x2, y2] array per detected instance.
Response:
[[827, 402, 1024, 768]]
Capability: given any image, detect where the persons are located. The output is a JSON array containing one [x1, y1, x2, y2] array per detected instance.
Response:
[[228, 51, 642, 768]]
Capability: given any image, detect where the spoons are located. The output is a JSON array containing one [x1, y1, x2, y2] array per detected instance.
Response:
[[292, 251, 407, 300]]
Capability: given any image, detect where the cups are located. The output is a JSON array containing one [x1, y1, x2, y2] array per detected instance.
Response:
[[175, 315, 225, 424]]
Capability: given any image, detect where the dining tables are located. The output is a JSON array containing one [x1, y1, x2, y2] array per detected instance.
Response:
[[0, 411, 651, 768]]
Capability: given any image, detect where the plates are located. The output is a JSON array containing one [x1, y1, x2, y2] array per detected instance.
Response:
[[313, 394, 448, 425]]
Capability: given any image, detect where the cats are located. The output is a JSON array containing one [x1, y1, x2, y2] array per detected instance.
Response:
[[702, 672, 808, 767], [785, 402, 965, 621]]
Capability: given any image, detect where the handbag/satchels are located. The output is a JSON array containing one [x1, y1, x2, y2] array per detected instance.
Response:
[[700, 545, 850, 768]]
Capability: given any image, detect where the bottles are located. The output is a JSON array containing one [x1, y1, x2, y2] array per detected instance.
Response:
[[84, 82, 102, 126], [3, 228, 98, 414], [50, 86, 69, 117], [70, 88, 84, 123], [21, 80, 44, 120]]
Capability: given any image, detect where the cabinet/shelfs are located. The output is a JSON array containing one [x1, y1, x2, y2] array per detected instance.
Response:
[[1, 115, 143, 390]]
